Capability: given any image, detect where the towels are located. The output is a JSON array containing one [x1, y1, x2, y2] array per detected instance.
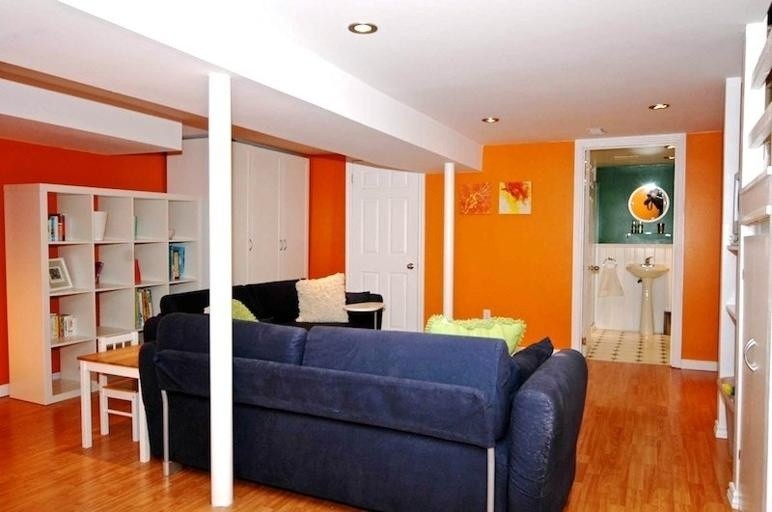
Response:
[[598, 266, 625, 299]]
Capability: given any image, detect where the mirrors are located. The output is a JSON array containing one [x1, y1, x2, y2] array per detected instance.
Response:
[[626, 181, 670, 224]]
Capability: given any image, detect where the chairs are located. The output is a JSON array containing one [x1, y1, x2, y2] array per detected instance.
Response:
[[97, 330, 139, 443]]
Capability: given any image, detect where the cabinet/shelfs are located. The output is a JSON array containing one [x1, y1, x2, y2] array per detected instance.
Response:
[[2, 182, 202, 405], [166, 135, 310, 290], [711, 10, 772, 512]]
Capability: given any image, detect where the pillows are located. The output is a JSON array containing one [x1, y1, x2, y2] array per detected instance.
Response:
[[232, 297, 259, 322], [422, 311, 528, 355], [295, 270, 351, 324]]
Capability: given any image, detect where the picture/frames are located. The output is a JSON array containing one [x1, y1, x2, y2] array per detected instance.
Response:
[[46, 256, 74, 292]]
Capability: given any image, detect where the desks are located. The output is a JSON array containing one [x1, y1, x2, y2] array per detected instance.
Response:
[[76, 343, 152, 463], [342, 300, 385, 330]]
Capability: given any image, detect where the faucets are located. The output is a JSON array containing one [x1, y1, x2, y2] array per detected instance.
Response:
[[642, 255, 656, 268]]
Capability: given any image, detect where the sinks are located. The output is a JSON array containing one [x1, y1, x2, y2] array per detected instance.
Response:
[[626, 263, 669, 279]]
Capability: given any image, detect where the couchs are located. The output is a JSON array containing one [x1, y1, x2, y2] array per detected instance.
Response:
[[137, 277, 590, 511]]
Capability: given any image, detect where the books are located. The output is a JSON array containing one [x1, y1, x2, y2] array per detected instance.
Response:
[[48, 213, 67, 242], [169, 245, 186, 283], [135, 259, 140, 285], [51, 311, 77, 340], [136, 288, 153, 330], [95, 262, 104, 285]]
